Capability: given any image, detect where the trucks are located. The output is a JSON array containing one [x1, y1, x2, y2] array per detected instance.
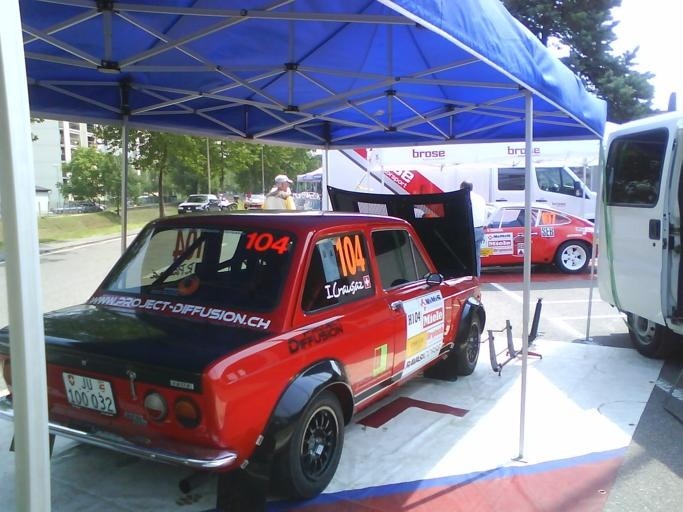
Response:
[[322, 148, 597, 223]]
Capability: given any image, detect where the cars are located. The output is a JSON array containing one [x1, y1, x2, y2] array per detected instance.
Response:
[[479, 202, 598, 273]]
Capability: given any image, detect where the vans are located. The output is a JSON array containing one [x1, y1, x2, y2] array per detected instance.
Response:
[[598, 109, 682, 358]]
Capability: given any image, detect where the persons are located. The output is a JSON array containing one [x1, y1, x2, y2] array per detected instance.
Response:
[[260, 173, 295, 209]]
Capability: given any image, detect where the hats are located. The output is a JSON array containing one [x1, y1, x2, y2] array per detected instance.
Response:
[[274, 174, 292, 184]]
